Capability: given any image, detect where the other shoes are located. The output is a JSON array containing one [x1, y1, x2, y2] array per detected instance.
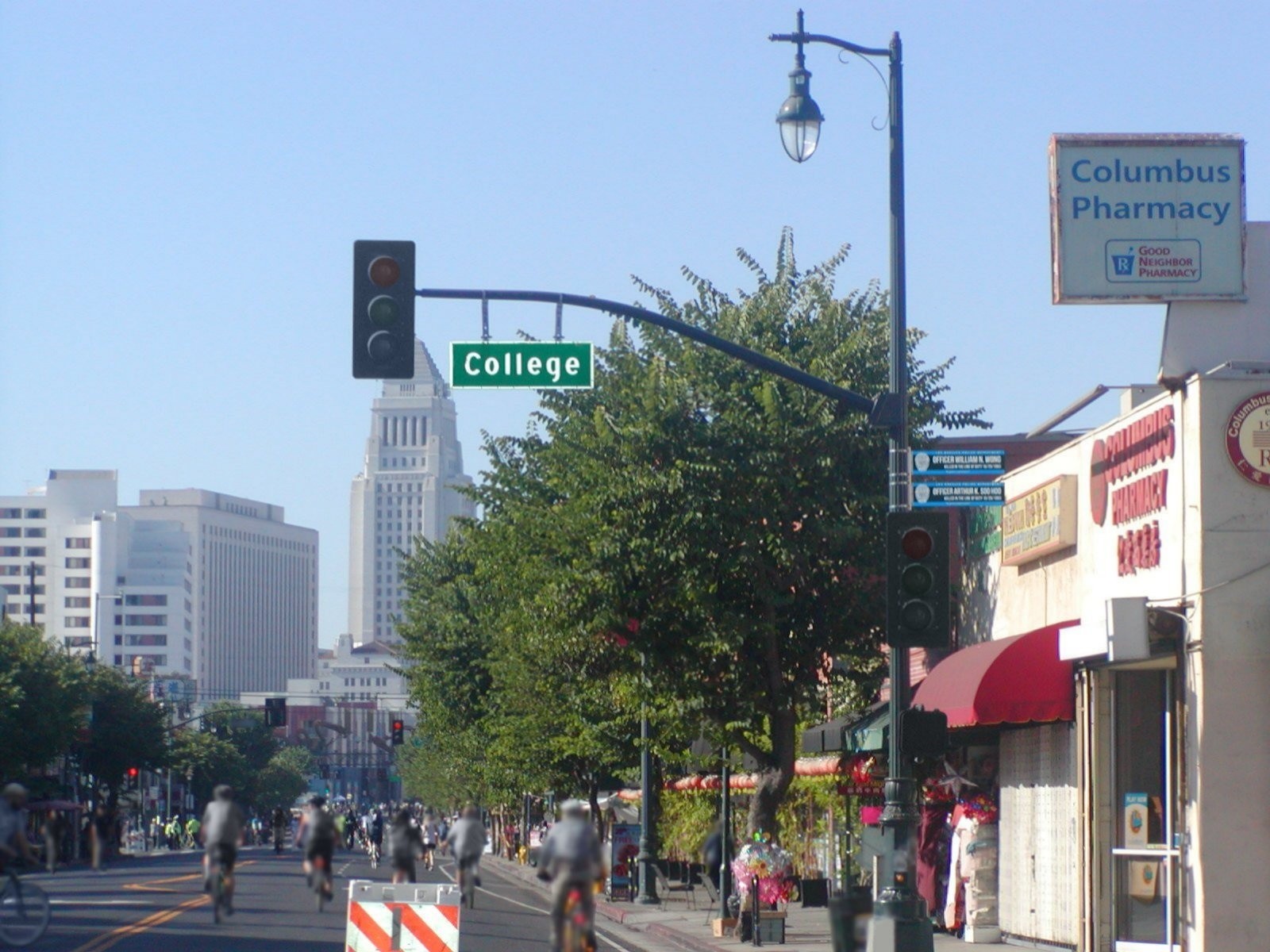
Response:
[[473, 875, 481, 887], [460, 892, 466, 904], [424, 863, 428, 869], [325, 890, 334, 901], [307, 872, 314, 888], [429, 866, 434, 871]]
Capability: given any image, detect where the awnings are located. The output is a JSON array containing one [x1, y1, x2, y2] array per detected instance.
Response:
[[910, 618, 1082, 727], [803, 699, 889, 754]]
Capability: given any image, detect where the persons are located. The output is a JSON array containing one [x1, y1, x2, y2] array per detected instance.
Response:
[[291, 795, 344, 903], [533, 799, 612, 952], [702, 820, 738, 902], [198, 784, 246, 916], [0, 782, 42, 877], [439, 801, 490, 905], [388, 807, 426, 886], [148, 796, 555, 852]]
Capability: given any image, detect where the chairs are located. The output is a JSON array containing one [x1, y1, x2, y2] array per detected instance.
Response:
[[648, 863, 698, 913], [695, 873, 720, 926]]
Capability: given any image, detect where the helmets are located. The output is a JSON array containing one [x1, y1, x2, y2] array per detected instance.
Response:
[[305, 795, 324, 806]]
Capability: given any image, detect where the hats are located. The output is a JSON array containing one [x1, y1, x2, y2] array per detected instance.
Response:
[[561, 799, 583, 812], [166, 818, 173, 821], [96, 800, 106, 807], [173, 815, 179, 820]]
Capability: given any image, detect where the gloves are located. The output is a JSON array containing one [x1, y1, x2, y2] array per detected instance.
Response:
[[536, 872, 552, 882]]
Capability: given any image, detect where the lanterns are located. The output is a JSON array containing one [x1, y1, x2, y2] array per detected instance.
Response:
[[616, 788, 643, 807], [657, 771, 764, 799], [790, 752, 845, 785]]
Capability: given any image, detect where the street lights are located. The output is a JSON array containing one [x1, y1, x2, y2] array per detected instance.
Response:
[[768, 7, 938, 952]]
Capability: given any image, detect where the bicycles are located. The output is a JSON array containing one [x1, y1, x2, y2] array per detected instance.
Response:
[[437, 842, 488, 909], [354, 829, 454, 883], [196, 839, 243, 925], [0, 846, 52, 950], [293, 838, 348, 915], [535, 869, 604, 952]]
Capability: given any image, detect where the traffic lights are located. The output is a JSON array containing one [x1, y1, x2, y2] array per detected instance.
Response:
[[884, 510, 952, 649], [352, 239, 417, 380], [127, 767, 138, 790], [392, 719, 403, 745]]
